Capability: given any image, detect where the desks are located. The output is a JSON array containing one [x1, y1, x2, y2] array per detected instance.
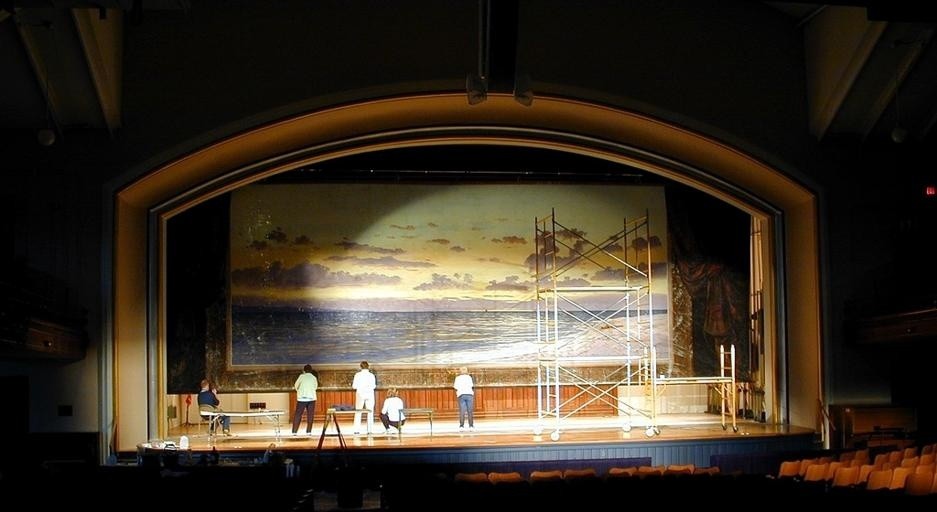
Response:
[[330, 409, 372, 434], [199, 410, 284, 438]]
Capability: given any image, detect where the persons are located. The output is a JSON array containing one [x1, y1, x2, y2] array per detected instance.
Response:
[[351, 361, 408, 437], [292, 364, 318, 435], [197, 379, 230, 436], [453, 365, 478, 431]]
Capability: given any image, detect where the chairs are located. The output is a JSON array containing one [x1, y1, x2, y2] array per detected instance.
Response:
[[455, 439, 936, 511], [199, 403, 224, 436]]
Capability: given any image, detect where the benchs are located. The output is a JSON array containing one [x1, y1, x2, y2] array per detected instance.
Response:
[[398, 408, 434, 435]]
